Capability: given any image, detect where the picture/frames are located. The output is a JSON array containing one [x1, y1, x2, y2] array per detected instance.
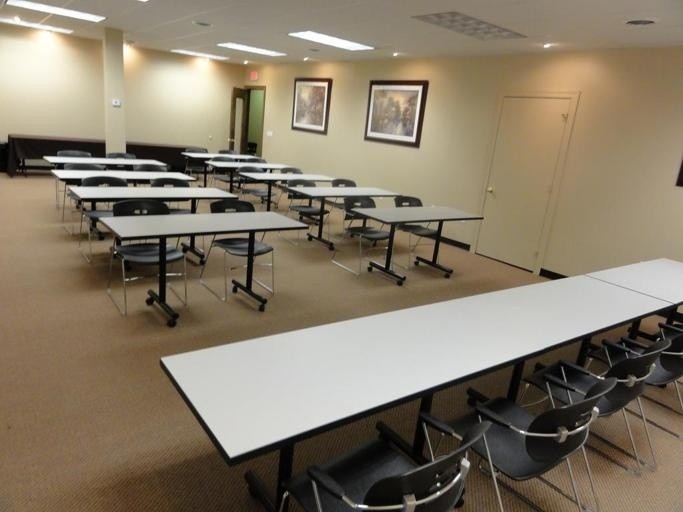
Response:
[[364, 80, 429, 149], [291, 78, 332, 133]]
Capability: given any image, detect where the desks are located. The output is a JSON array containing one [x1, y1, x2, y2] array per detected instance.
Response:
[[9, 133, 208, 178]]
